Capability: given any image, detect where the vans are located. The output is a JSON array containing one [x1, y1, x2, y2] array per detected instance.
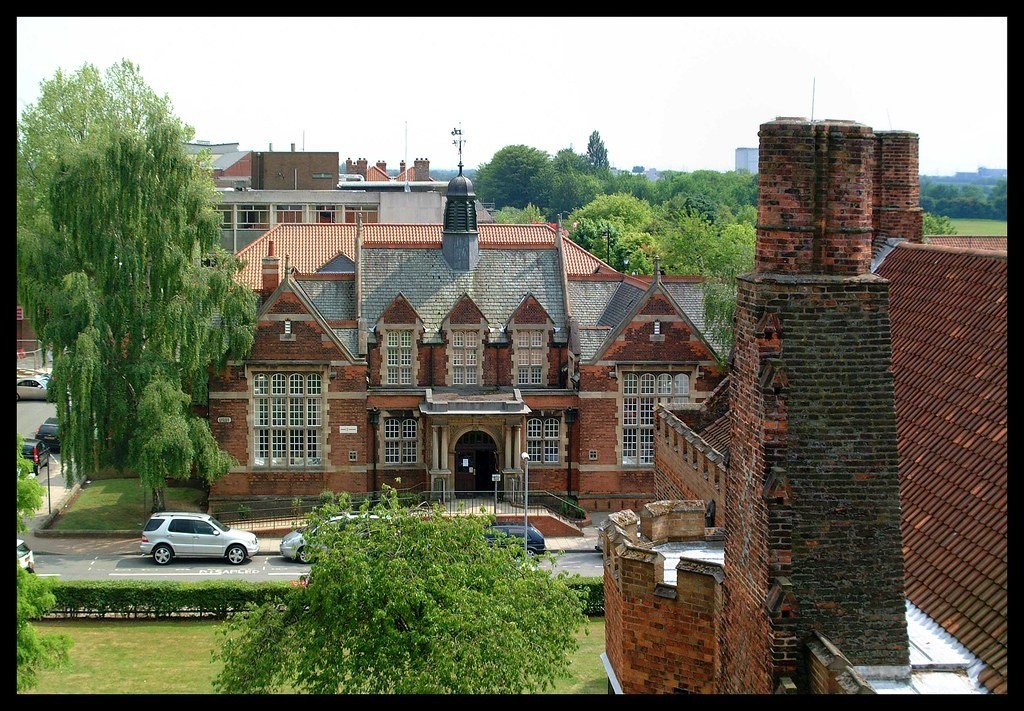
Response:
[[280, 515, 392, 564]]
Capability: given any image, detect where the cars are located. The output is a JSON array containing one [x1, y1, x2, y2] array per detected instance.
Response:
[[20, 437, 48, 476], [17, 539, 34, 570], [16, 377, 51, 402], [594, 521, 641, 552], [481, 521, 546, 561]]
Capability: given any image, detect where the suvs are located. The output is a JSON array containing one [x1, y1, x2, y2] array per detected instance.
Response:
[[140, 513, 260, 565], [35, 416, 62, 454]]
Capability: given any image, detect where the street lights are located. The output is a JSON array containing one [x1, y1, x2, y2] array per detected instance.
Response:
[[521, 452, 530, 552], [564, 406, 575, 497], [367, 406, 378, 509]]
